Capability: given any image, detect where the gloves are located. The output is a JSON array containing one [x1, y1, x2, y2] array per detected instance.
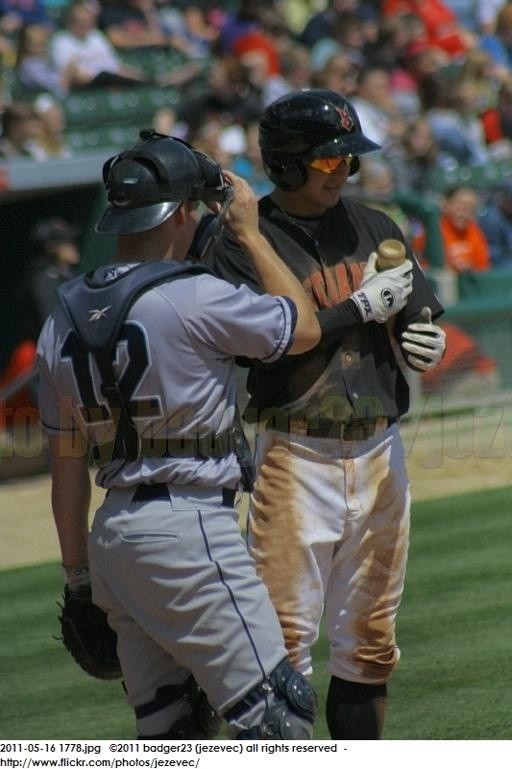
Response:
[[398, 304, 448, 377], [348, 251, 415, 326]]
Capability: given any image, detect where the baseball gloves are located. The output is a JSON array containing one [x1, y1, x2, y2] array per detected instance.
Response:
[[62, 554, 122, 678]]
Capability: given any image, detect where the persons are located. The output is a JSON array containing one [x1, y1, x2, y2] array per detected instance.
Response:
[[38, 127, 323, 738], [194, 89, 450, 740], [1, 0, 510, 435]]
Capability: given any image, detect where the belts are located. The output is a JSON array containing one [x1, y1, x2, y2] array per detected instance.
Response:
[[266, 416, 398, 446]]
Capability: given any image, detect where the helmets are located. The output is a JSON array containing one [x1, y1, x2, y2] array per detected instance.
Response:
[[257, 86, 384, 193], [92, 127, 235, 239]]
